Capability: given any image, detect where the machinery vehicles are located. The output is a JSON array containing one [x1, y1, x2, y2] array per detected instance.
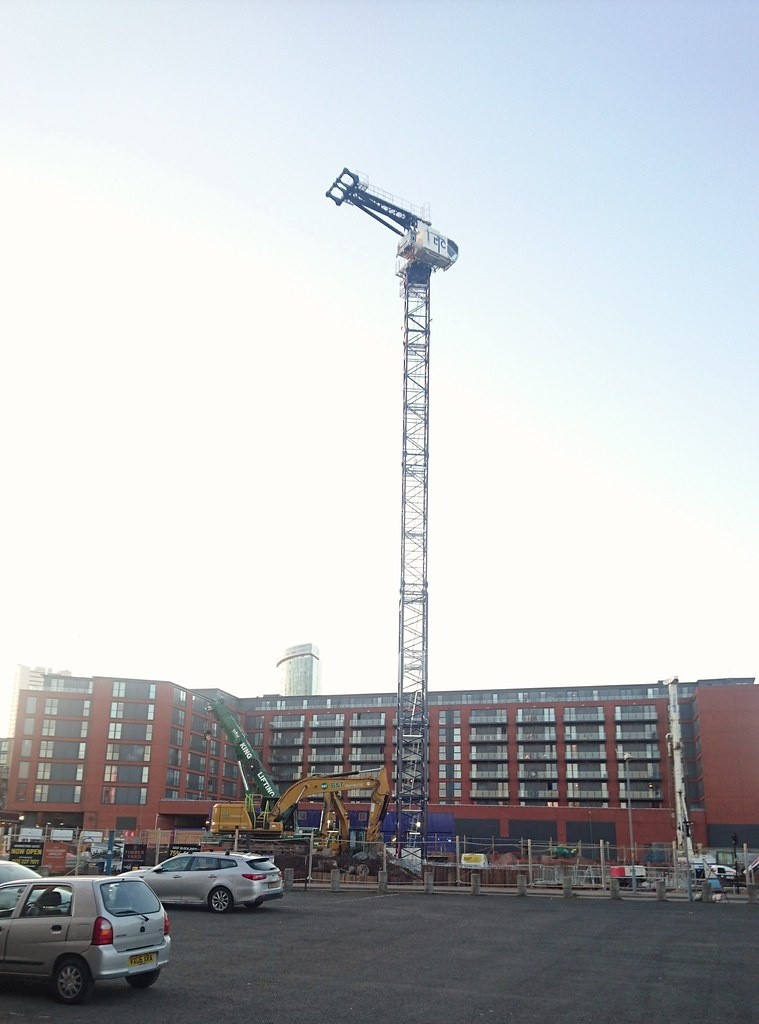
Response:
[[206, 696, 303, 836], [202, 764, 393, 878], [661, 675, 738, 883]]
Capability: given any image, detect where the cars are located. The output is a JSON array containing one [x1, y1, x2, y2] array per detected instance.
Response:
[[0, 876, 172, 1004], [0, 859, 72, 914]]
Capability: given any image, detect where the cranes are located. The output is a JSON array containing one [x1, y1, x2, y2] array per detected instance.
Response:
[[324, 167, 461, 876]]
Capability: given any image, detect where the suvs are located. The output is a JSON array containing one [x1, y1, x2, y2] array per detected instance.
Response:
[[109, 850, 284, 914]]
